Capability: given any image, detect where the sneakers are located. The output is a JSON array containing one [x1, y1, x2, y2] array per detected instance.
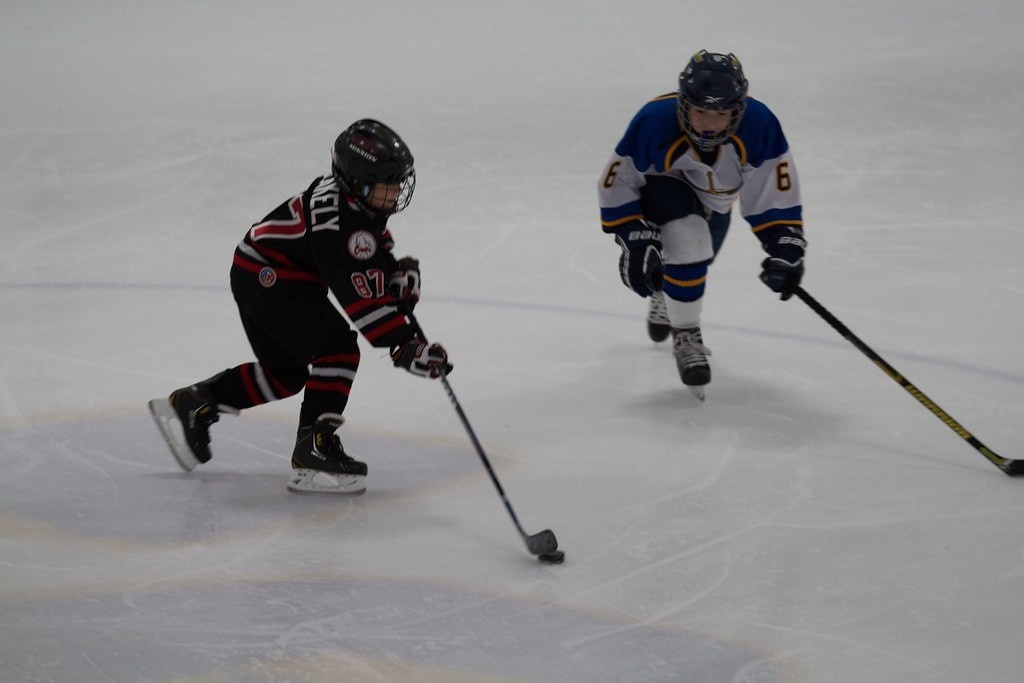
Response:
[[647, 290, 670, 342], [670, 323, 712, 401], [148, 369, 240, 471], [287, 403, 368, 494]]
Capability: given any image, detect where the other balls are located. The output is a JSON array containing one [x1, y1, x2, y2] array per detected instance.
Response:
[[539, 551, 564, 564]]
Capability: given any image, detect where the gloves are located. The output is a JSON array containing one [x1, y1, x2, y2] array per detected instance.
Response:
[[392, 339, 453, 380], [760, 234, 807, 300], [388, 257, 421, 315], [615, 220, 664, 298]]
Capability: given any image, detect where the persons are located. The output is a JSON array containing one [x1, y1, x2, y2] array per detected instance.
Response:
[[599, 49, 807, 400], [148, 118, 453, 496]]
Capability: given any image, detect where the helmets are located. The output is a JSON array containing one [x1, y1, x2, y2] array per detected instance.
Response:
[[332, 118, 414, 184], [679, 49, 748, 110]]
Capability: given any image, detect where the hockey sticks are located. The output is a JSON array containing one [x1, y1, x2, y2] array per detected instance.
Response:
[[407, 305, 558, 556], [760, 255, 1024, 481]]
